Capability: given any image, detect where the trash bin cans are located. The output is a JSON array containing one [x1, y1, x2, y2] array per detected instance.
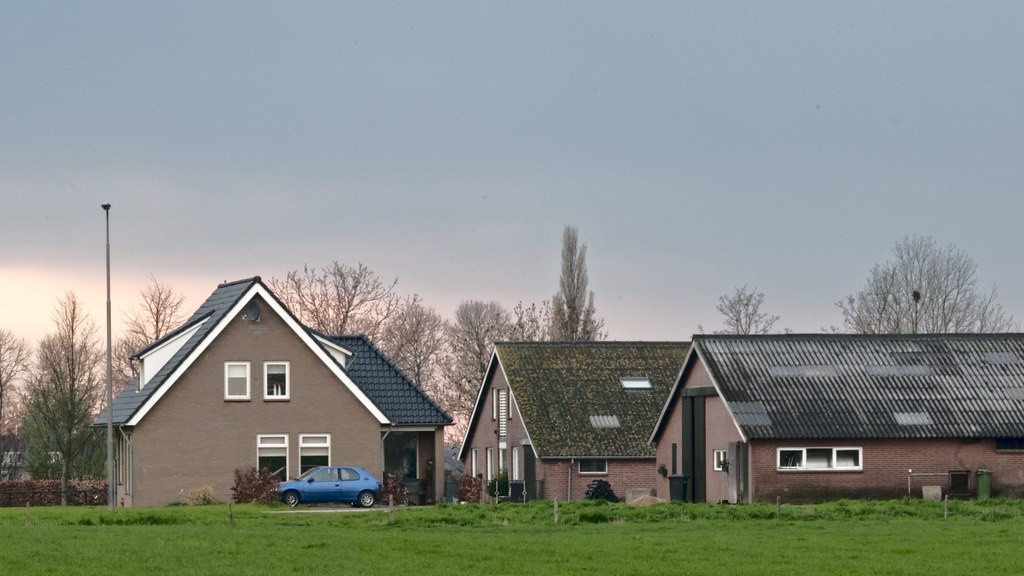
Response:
[[975, 468, 994, 502], [670, 475, 689, 504], [510, 482, 525, 501]]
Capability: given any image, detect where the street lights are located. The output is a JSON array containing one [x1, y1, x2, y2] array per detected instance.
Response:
[[99, 200, 115, 510]]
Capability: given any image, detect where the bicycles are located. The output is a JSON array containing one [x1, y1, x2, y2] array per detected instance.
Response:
[[402, 485, 409, 506]]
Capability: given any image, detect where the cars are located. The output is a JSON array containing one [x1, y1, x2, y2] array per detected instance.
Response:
[[270, 465, 381, 511]]
